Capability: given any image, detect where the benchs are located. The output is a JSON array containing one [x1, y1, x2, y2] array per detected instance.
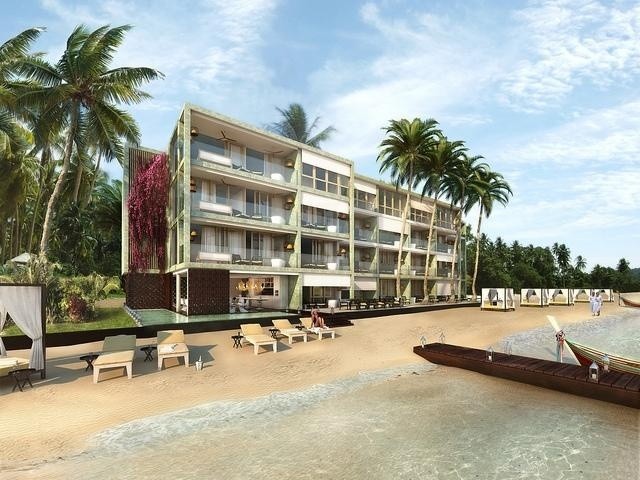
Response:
[[196, 252, 233, 263], [197, 148, 232, 168], [199, 200, 233, 216]]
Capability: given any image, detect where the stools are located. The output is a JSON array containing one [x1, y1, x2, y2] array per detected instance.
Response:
[[80, 355, 98, 371], [232, 335, 243, 349], [8, 368, 36, 392], [140, 347, 157, 361]]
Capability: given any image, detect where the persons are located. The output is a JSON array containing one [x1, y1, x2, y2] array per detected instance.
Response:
[[309, 311, 324, 328], [590, 292, 603, 316]]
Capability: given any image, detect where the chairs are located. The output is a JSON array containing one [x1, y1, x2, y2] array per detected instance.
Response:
[[233, 164, 264, 176], [239, 317, 335, 355], [232, 254, 263, 266], [156, 330, 189, 371], [92, 334, 136, 384], [301, 220, 326, 230], [301, 260, 326, 269], [303, 295, 468, 310], [233, 209, 263, 220]]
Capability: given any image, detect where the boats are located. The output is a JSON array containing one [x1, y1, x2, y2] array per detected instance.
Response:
[[563, 338, 640, 376], [621, 296, 640, 307]]
